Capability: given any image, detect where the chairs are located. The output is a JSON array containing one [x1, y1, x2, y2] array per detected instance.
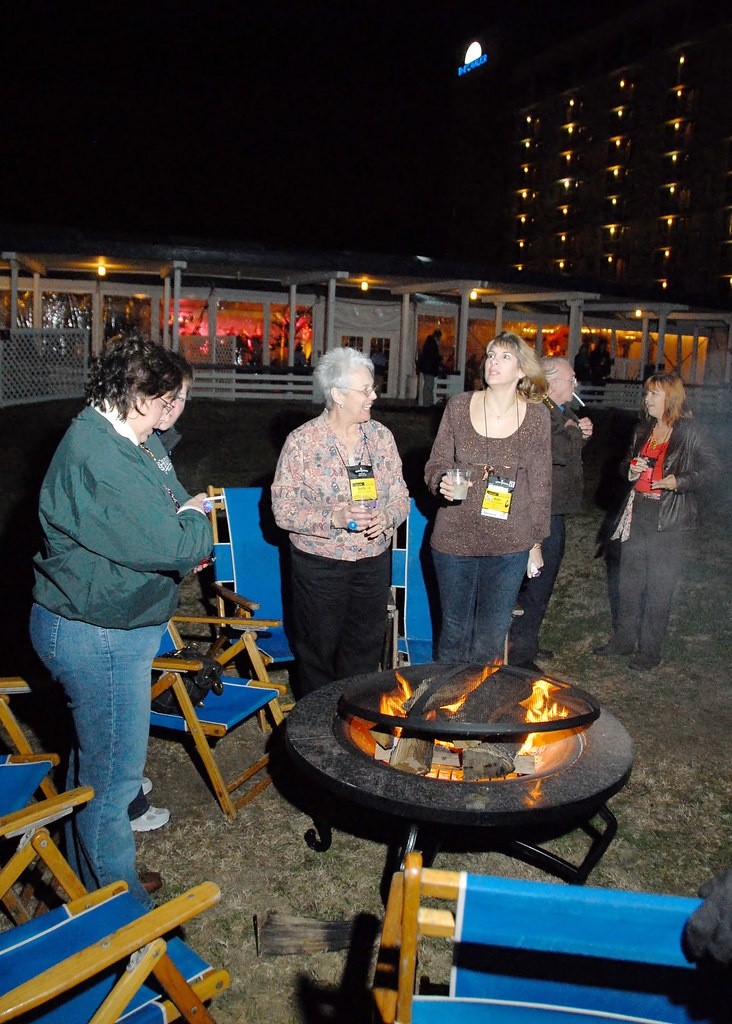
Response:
[[148, 487, 513, 820], [357, 851, 708, 1024], [0, 675, 231, 1024]]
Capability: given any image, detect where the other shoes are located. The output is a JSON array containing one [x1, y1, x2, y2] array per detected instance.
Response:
[[136, 871, 161, 894], [629, 658, 660, 671], [594, 643, 635, 656]]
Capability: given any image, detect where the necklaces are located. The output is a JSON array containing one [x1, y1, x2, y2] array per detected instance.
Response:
[[486, 394, 516, 423], [352, 424, 377, 509], [140, 442, 208, 565], [649, 424, 664, 450]]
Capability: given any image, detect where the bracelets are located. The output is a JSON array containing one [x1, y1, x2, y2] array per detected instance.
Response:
[[533, 544, 541, 549]]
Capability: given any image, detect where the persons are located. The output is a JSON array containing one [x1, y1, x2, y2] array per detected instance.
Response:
[[178, 314, 201, 336], [416, 328, 447, 408], [590, 335, 611, 402], [424, 330, 558, 663], [465, 352, 477, 375], [508, 355, 593, 674], [594, 368, 727, 671], [573, 333, 593, 400], [345, 337, 388, 373], [235, 333, 307, 367], [28, 334, 215, 911], [271, 347, 411, 696], [126, 348, 194, 830]]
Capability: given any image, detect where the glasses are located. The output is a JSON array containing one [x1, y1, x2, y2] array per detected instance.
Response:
[[338, 384, 379, 397], [552, 376, 577, 387], [155, 393, 174, 415]]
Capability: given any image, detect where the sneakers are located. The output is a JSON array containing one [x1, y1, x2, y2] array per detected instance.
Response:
[[130, 805, 171, 831], [142, 777, 153, 795]]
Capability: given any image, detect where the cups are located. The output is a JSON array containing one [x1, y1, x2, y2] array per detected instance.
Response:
[[351, 495, 374, 516], [445, 468, 472, 501]]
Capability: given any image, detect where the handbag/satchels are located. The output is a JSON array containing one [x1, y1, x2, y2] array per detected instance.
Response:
[[155, 647, 223, 714]]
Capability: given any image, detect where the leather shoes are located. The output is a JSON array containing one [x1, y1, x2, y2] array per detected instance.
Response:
[[535, 648, 554, 660], [516, 662, 544, 674]]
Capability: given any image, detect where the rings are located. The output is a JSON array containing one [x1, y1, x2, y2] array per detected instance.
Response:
[[348, 520, 357, 531], [203, 500, 213, 514], [631, 459, 638, 465]]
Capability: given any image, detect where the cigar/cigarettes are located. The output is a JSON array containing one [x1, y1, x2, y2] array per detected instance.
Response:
[[572, 391, 585, 407]]
